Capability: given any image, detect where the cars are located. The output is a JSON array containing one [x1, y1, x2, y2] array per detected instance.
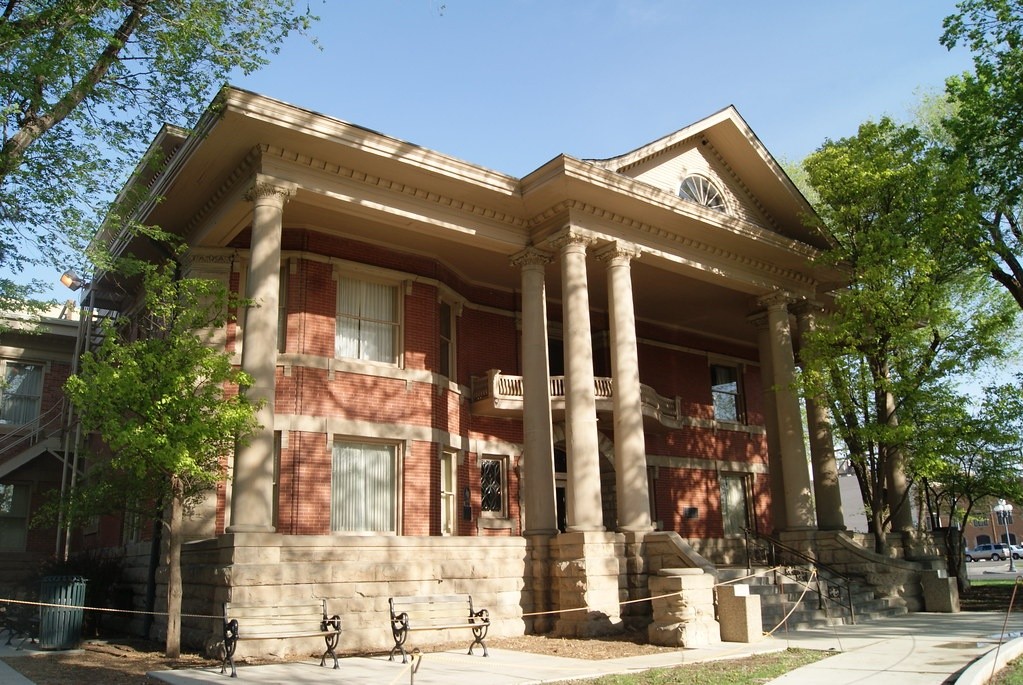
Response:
[[1008, 544, 1023, 559]]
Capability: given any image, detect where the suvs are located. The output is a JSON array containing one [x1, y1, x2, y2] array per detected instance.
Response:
[[965, 543, 1010, 562]]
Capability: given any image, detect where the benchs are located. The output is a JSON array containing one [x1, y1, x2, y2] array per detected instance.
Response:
[[385, 592, 490, 665], [220, 596, 341, 677], [0, 604, 41, 651]]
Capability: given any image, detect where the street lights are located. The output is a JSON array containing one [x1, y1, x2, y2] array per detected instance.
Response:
[[994, 498, 1017, 573], [54, 269, 88, 565]]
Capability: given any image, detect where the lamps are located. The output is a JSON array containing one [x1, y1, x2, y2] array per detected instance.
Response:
[[60, 270, 89, 290]]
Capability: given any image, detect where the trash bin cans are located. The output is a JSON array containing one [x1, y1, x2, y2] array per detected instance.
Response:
[[39, 575, 91, 648]]
